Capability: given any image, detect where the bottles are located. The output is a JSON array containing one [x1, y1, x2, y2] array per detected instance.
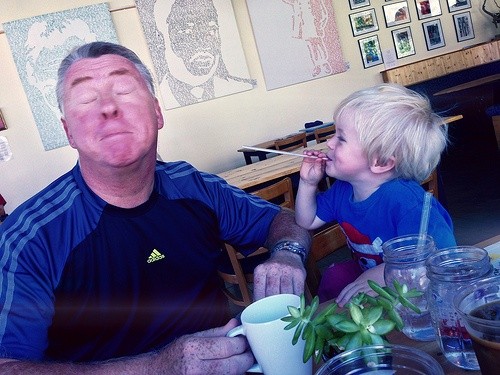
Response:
[[425, 245, 495, 370], [382, 234, 438, 342]]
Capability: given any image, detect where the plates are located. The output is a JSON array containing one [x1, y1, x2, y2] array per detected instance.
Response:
[[299, 122, 334, 133]]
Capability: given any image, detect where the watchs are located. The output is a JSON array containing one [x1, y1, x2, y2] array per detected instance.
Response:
[[271, 239, 308, 262]]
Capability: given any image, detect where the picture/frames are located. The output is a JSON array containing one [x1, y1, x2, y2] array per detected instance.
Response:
[[348, 0, 371, 10], [349, 8, 380, 37], [447, 0, 472, 12], [357, 34, 384, 69], [415, 0, 442, 20], [391, 26, 416, 59], [382, 0, 411, 28], [452, 11, 475, 42], [422, 18, 446, 51]]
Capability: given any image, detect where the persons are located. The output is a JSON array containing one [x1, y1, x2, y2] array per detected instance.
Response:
[[294, 83, 457, 304], [0, 41, 309, 374]]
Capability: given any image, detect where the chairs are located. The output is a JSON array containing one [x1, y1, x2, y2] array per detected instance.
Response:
[[217, 115, 463, 193], [298, 223, 347, 309], [314, 124, 336, 144], [214, 177, 295, 310], [274, 132, 307, 155]]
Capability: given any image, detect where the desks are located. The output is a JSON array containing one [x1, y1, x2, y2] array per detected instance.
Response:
[[237, 121, 336, 164], [433, 74, 500, 96]]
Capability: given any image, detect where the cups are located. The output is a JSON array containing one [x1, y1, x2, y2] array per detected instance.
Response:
[[315, 345, 445, 375], [227, 294, 313, 375], [453, 275, 500, 375]]
